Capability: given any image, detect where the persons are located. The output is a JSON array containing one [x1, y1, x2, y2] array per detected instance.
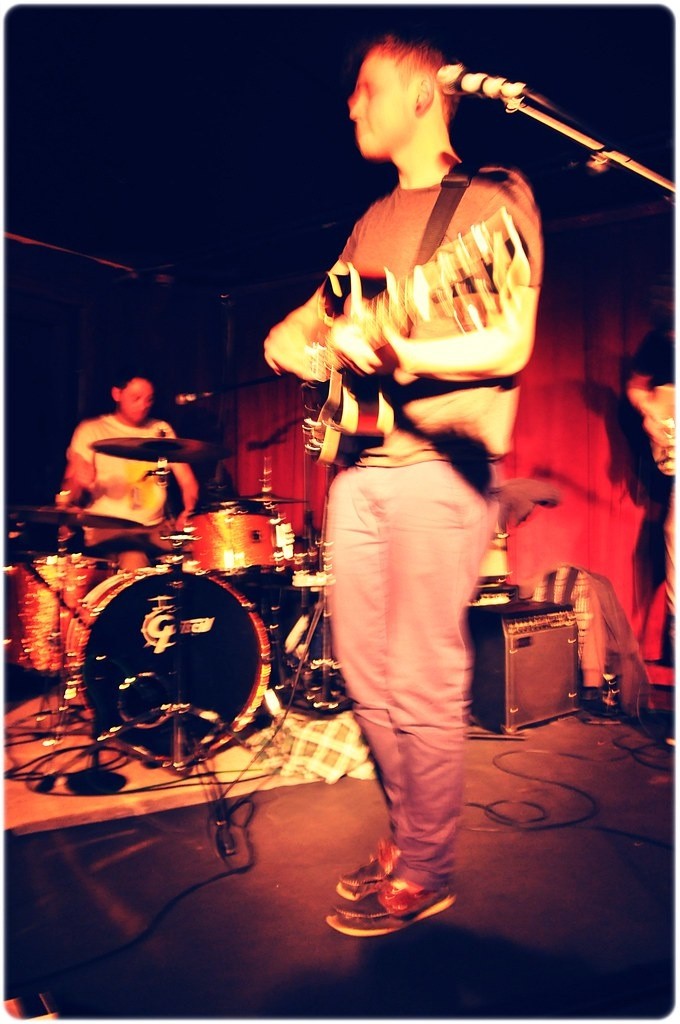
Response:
[[260, 33, 544, 937], [626, 275, 674, 751], [59, 369, 202, 571]]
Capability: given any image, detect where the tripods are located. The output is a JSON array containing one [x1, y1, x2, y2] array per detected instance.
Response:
[[5, 456, 262, 792]]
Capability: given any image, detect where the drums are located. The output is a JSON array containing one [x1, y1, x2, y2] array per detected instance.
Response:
[[3, 500, 294, 773]]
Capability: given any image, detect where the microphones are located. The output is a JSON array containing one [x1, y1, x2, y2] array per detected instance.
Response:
[[437, 64, 529, 100]]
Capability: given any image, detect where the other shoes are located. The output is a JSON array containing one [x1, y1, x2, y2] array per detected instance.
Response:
[[326, 876, 458, 937], [335, 832, 401, 900]]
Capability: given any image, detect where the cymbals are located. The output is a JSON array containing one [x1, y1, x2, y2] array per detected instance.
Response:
[[88, 438, 232, 464], [8, 507, 145, 531], [236, 492, 314, 506]]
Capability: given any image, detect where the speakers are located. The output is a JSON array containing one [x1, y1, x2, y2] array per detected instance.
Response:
[[466, 600, 583, 735]]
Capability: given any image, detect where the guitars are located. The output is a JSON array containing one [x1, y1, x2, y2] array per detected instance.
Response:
[[302, 202, 533, 469]]
